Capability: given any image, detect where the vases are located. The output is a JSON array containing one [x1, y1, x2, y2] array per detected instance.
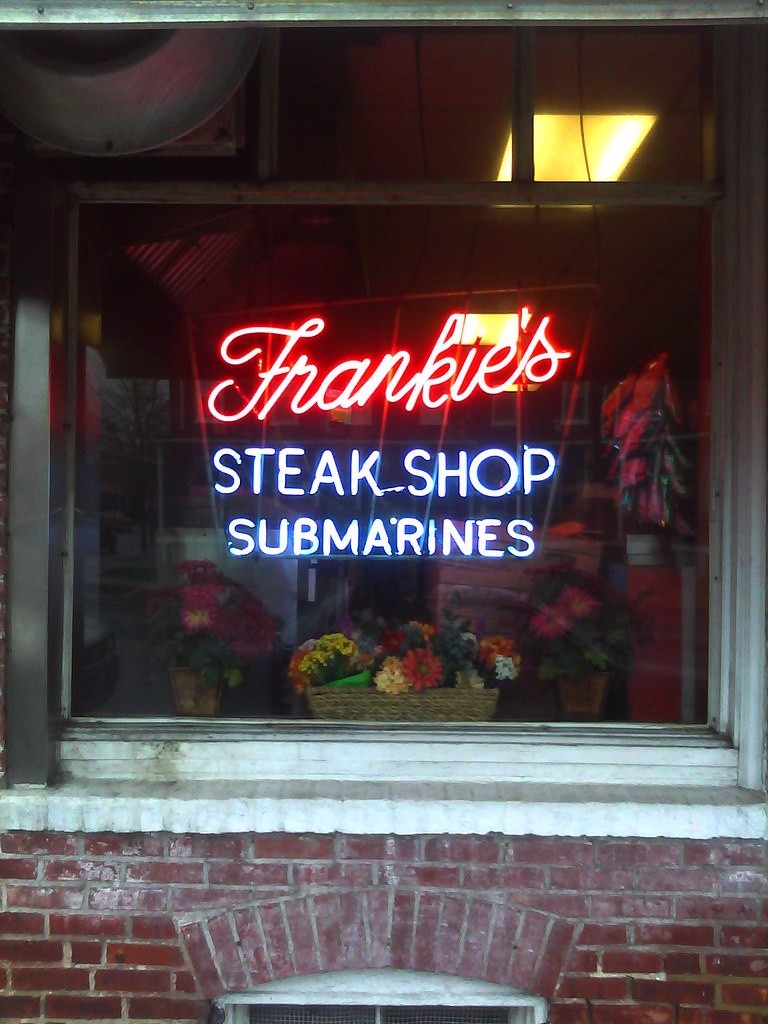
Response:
[[168, 666, 225, 717], [304, 686, 500, 721], [554, 670, 617, 721]]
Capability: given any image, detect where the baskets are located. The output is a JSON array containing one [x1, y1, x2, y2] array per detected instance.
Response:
[[305, 678, 498, 721]]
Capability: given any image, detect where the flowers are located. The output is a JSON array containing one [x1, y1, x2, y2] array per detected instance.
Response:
[[149, 560, 278, 687], [523, 560, 657, 685], [288, 616, 524, 696]]
